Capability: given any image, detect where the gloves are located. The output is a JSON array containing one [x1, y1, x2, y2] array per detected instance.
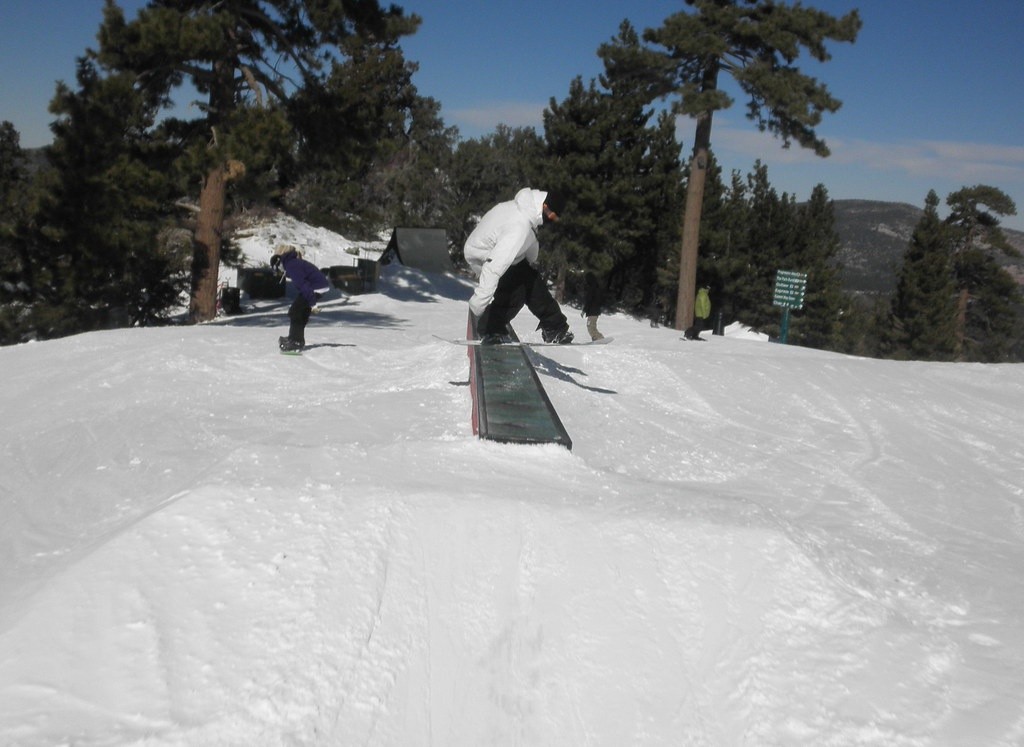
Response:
[[311, 305, 321, 315]]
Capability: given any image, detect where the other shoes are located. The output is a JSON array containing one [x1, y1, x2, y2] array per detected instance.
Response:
[[477, 331, 513, 343], [543, 329, 574, 343], [279, 336, 305, 351]]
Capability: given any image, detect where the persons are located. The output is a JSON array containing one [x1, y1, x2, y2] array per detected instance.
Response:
[[462, 186, 576, 345], [580, 282, 603, 341], [683, 284, 711, 341], [268, 250, 330, 353]]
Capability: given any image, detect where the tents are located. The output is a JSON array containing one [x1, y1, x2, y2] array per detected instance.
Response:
[[377, 226, 455, 272]]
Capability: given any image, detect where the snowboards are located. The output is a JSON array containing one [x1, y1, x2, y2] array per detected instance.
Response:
[[280, 349, 302, 356], [432, 334, 615, 347], [679, 337, 705, 341]]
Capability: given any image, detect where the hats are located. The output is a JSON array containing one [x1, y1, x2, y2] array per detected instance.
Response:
[[270, 255, 281, 272], [546, 192, 563, 216]]
